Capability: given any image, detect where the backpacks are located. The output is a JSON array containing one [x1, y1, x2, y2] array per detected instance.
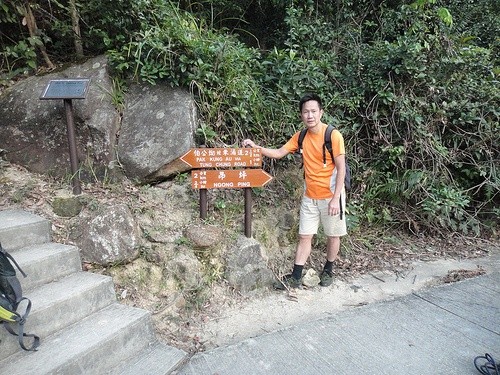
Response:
[[297, 124, 351, 191], [0, 242, 40, 352]]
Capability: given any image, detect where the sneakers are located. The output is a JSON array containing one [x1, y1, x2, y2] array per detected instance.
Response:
[[273, 276, 302, 289], [320, 269, 333, 286]]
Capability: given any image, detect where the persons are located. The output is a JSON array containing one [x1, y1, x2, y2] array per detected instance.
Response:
[[241, 94, 351, 290]]
